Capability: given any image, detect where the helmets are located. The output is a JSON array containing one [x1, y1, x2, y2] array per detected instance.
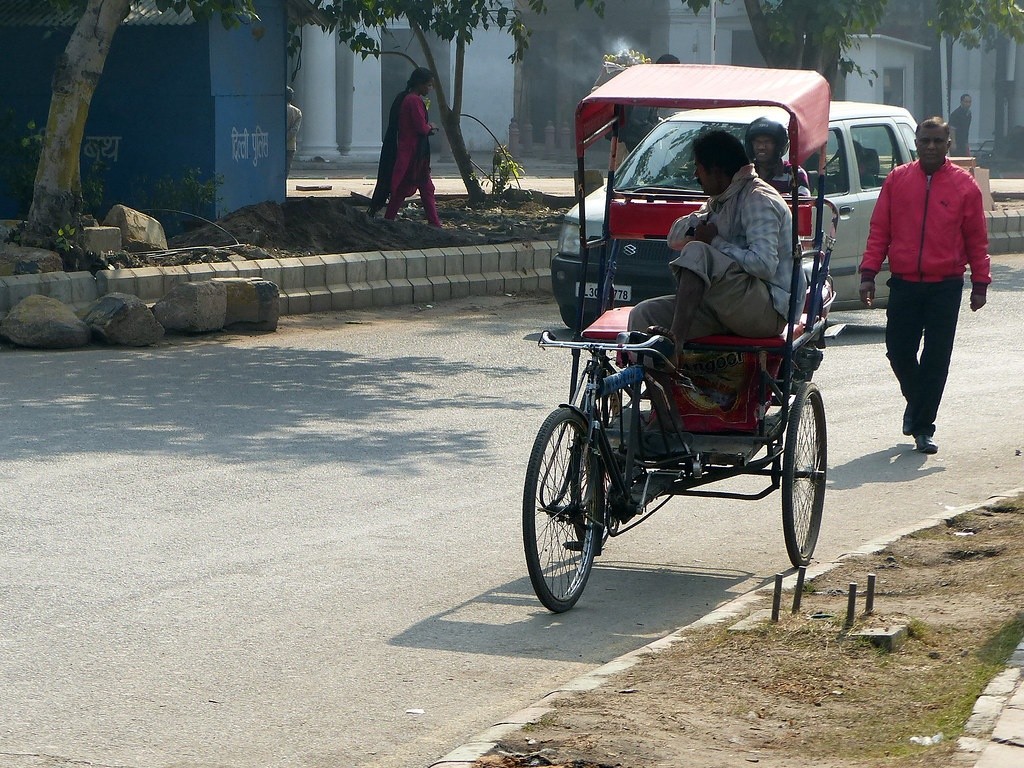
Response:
[[745, 115, 789, 163]]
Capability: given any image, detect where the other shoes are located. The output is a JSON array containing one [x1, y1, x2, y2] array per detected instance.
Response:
[[915, 435, 938, 454], [904, 400, 922, 435]]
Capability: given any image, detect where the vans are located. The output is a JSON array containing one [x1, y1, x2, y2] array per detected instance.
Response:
[[551, 101, 919, 329]]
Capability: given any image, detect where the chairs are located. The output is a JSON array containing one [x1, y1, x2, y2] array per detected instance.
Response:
[[863, 148, 886, 187]]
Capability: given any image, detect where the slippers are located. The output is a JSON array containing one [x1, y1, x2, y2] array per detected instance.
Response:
[[632, 430, 694, 459]]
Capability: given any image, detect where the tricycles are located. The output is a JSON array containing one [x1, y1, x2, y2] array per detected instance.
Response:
[[522, 63, 829, 612]]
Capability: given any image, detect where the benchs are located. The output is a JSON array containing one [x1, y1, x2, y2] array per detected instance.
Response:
[[579, 200, 815, 355]]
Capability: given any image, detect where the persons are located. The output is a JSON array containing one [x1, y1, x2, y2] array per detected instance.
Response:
[[366, 67, 441, 227], [857, 116, 993, 454], [948, 94, 973, 157], [287, 85, 303, 177], [617, 129, 808, 461], [604, 54, 681, 153], [743, 117, 825, 285]]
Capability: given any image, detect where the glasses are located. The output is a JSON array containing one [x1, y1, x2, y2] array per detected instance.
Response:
[[914, 137, 949, 147]]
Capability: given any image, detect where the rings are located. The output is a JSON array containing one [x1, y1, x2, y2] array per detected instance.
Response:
[[694, 229, 697, 231], [707, 221, 712, 225]]
